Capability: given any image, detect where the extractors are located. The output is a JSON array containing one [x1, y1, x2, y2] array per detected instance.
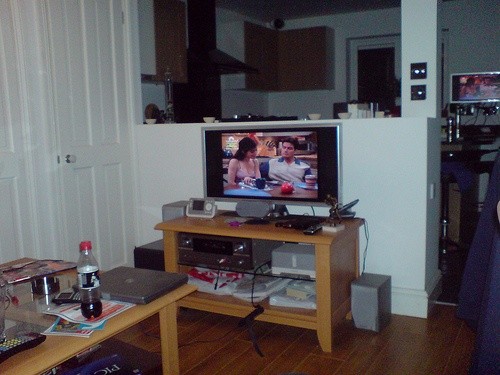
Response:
[[217, 48, 252, 75]]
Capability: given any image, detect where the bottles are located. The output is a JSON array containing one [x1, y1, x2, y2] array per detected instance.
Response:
[[76, 240, 104, 319], [224, 149, 233, 159]]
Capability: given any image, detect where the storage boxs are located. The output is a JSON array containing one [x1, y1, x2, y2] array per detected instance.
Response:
[[271, 243, 316, 280]]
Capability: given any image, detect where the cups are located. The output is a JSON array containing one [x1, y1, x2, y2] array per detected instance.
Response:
[[0, 282, 6, 342], [256, 177, 266, 190], [304, 174, 318, 187], [281, 180, 293, 193]]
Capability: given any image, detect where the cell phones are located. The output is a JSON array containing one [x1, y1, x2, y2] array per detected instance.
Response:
[[53, 291, 82, 303], [302, 224, 322, 234]]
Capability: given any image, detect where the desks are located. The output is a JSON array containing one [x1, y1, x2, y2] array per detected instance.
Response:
[[0, 266, 200, 375]]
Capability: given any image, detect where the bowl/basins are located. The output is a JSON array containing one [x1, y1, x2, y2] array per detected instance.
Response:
[[307, 113, 322, 120], [31, 277, 60, 295], [203, 117, 215, 123], [144, 118, 157, 124], [337, 112, 352, 119]]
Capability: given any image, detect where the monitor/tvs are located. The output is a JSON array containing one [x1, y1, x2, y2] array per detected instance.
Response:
[[201, 122, 343, 207], [449, 72, 500, 103]]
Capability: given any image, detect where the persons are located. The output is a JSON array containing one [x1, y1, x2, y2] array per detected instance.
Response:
[[268, 138, 313, 185], [227, 137, 261, 186]]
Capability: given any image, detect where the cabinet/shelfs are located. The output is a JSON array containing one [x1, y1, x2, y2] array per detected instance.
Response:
[[155, 215, 365, 353], [137, 0, 188, 85], [244, 20, 336, 91]]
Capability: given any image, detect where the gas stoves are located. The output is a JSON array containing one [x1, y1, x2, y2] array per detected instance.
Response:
[[222, 113, 298, 122]]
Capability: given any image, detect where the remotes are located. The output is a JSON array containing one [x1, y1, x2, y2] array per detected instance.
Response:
[[0, 332, 47, 362]]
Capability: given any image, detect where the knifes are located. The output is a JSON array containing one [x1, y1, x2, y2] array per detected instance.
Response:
[[266, 140, 275, 148]]
